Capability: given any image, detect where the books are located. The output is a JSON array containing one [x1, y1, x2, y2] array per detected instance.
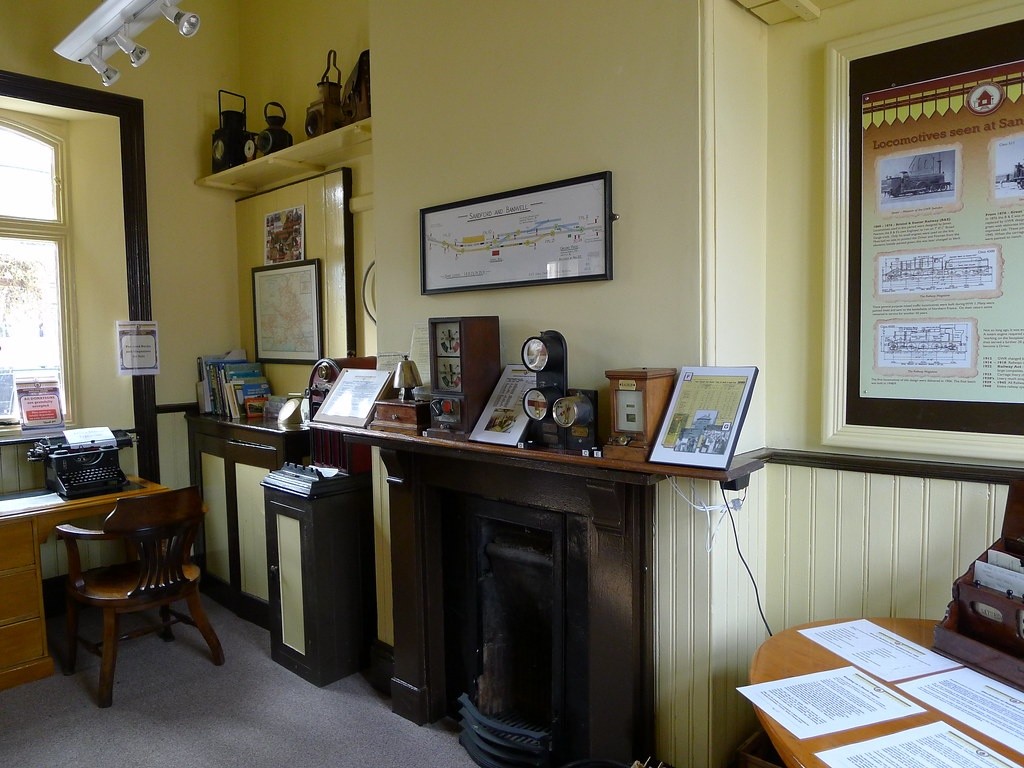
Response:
[[224, 362, 273, 419], [196, 351, 247, 419]]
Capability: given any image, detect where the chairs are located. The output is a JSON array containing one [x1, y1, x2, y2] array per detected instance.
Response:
[[54, 485, 225, 708]]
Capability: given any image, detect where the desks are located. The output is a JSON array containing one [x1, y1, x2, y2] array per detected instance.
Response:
[[749, 616, 1024, 768], [343, 433, 765, 768]]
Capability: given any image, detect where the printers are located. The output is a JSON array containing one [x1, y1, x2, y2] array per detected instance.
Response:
[[27, 427, 142, 501]]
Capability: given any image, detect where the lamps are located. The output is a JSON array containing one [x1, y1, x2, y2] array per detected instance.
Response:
[[112, 16, 150, 67], [160, 0, 200, 37], [87, 40, 121, 87]]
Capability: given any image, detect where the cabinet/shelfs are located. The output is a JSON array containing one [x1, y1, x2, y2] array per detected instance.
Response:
[[188, 421, 310, 630], [0, 516, 48, 670]]
